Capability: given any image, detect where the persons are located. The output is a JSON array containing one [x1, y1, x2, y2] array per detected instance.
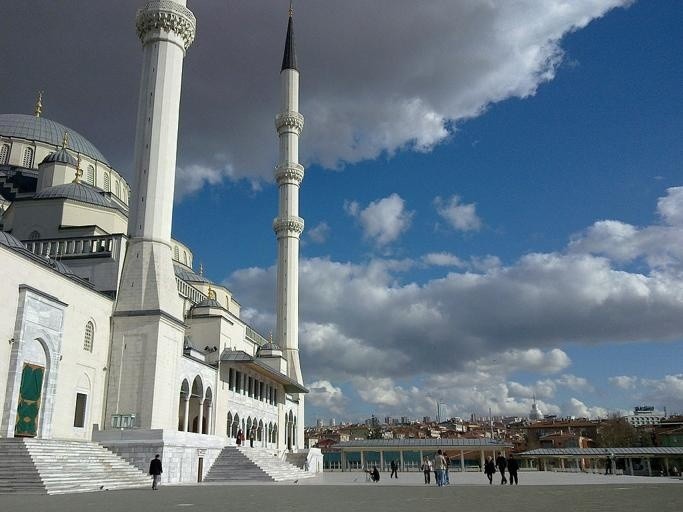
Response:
[[507, 454, 519, 485], [390, 459, 398, 478], [241, 434, 245, 446], [421, 449, 452, 487], [484, 456, 496, 484], [657, 464, 681, 477], [370, 466, 380, 482], [604, 456, 612, 474], [496, 452, 507, 484], [149, 454, 164, 490], [304, 458, 309, 471], [249, 431, 256, 447], [236, 429, 243, 447]]
